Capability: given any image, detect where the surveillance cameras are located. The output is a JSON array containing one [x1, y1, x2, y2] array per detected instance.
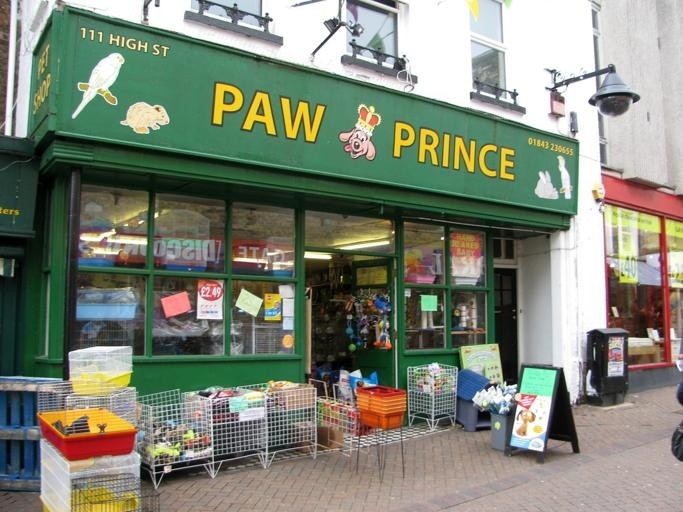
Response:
[[588, 64, 640, 118]]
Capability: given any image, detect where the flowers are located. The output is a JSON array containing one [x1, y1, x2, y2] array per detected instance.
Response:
[[471, 379, 517, 416]]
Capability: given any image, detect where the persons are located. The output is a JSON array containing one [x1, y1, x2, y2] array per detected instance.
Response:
[[676, 336, 683, 406]]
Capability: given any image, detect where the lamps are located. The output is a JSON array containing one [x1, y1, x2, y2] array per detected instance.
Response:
[[309, 15, 363, 57]]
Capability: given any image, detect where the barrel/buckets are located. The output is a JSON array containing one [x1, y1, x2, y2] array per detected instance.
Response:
[[660, 338, 682, 361]]
